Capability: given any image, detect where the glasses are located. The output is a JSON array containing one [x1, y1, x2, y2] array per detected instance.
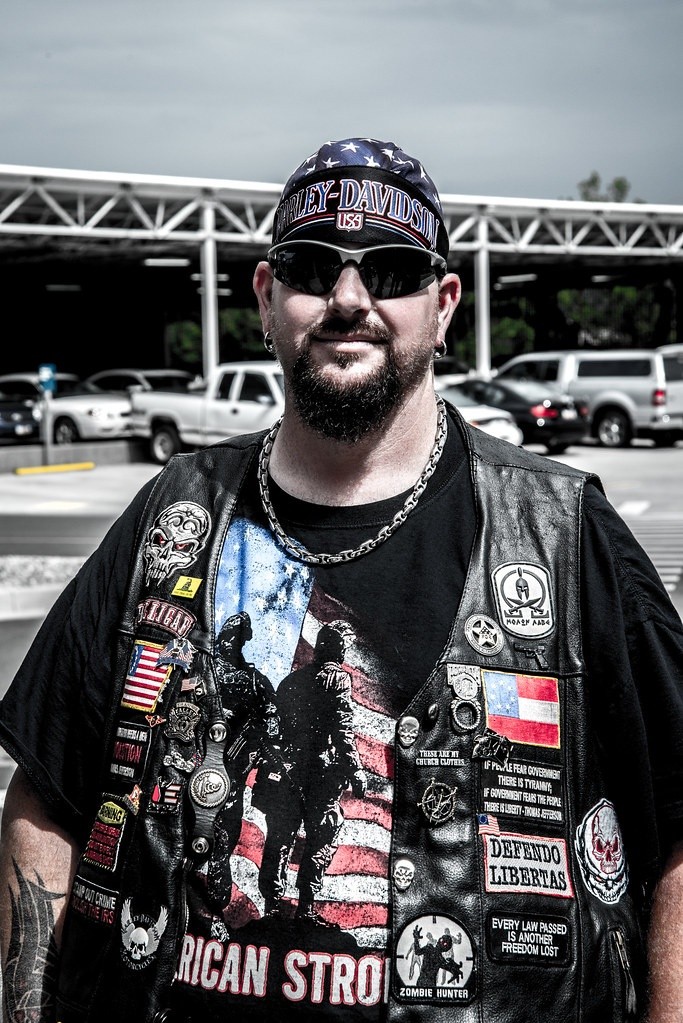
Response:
[[267, 240, 449, 298]]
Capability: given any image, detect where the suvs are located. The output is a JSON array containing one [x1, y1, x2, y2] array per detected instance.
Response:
[[496, 347, 683, 449]]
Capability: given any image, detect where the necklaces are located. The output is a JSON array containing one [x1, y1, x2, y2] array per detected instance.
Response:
[[257, 400, 447, 566]]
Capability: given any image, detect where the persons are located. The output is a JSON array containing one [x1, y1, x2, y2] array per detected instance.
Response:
[[205, 612, 280, 943], [254, 619, 366, 930], [1, 140, 682, 1023]]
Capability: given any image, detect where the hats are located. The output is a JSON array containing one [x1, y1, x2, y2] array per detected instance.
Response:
[[270, 138, 449, 262]]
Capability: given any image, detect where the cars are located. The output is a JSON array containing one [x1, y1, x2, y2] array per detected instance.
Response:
[[0, 392, 44, 445], [0, 372, 129, 444], [449, 378, 590, 451], [434, 389, 523, 447], [87, 369, 208, 401]]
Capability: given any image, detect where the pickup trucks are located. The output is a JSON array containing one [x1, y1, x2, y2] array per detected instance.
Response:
[[130, 363, 285, 462]]
